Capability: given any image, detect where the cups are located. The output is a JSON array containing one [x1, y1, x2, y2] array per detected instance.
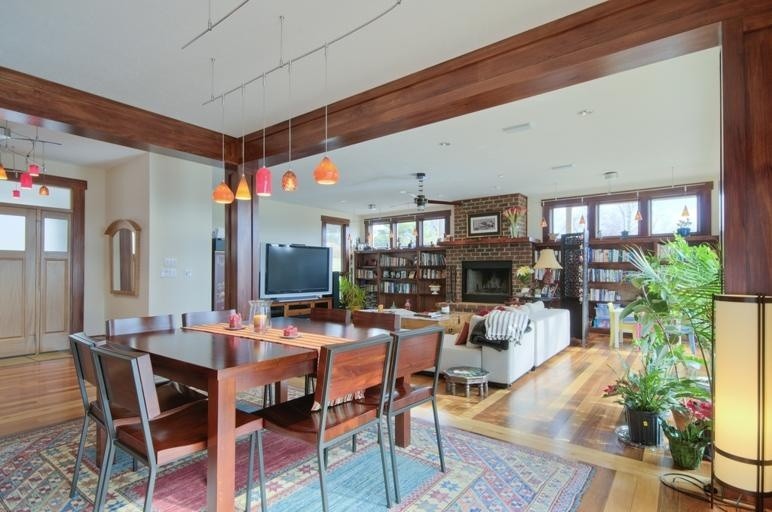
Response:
[[378, 304, 383, 313], [248, 300, 274, 332]]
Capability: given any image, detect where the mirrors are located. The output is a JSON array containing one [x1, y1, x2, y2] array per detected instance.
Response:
[[104, 219, 142, 297]]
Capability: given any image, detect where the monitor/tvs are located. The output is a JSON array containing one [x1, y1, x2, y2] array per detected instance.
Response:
[[259, 241, 334, 303]]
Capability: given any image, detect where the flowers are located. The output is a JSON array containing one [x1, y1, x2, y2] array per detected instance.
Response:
[[603, 330, 713, 469], [501, 206, 527, 225], [516, 266, 534, 287]]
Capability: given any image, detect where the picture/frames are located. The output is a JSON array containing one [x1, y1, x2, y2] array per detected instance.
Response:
[[466, 211, 502, 239]]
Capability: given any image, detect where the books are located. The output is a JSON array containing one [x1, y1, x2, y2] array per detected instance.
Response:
[[533, 249, 561, 282], [355, 253, 446, 294], [414, 311, 441, 318], [587, 241, 687, 304]]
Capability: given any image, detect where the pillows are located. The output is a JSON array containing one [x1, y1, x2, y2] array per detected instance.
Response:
[[454, 300, 544, 348]]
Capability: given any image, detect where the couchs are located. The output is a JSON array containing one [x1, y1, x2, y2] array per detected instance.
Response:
[[513, 308, 570, 367]]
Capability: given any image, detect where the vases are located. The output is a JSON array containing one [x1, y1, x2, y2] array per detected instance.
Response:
[[510, 224, 520, 238]]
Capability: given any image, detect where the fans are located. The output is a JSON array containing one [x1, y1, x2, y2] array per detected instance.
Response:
[[391, 172, 462, 211]]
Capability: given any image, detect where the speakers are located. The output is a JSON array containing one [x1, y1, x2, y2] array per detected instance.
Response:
[[332, 272, 340, 306]]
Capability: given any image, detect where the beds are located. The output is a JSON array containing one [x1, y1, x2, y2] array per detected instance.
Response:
[[422, 321, 535, 388]]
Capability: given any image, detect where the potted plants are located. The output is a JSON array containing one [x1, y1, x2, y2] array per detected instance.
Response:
[[619, 239, 720, 431], [677, 219, 692, 236]]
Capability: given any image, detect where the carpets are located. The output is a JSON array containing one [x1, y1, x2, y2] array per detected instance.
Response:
[[0, 385, 597, 512]]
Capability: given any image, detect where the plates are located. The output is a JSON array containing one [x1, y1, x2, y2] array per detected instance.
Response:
[[224, 326, 245, 330], [280, 333, 301, 339]]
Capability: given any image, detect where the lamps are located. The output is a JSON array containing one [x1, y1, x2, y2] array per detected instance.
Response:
[[201, 0, 403, 204], [0, 120, 62, 199], [533, 248, 563, 287], [346, 211, 424, 240], [710, 294, 772, 512], [535, 167, 706, 228]]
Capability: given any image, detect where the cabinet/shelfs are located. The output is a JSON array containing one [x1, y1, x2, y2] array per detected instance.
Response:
[[519, 297, 581, 341], [588, 236, 719, 333], [354, 248, 446, 311], [533, 243, 562, 290], [267, 297, 334, 318]]
